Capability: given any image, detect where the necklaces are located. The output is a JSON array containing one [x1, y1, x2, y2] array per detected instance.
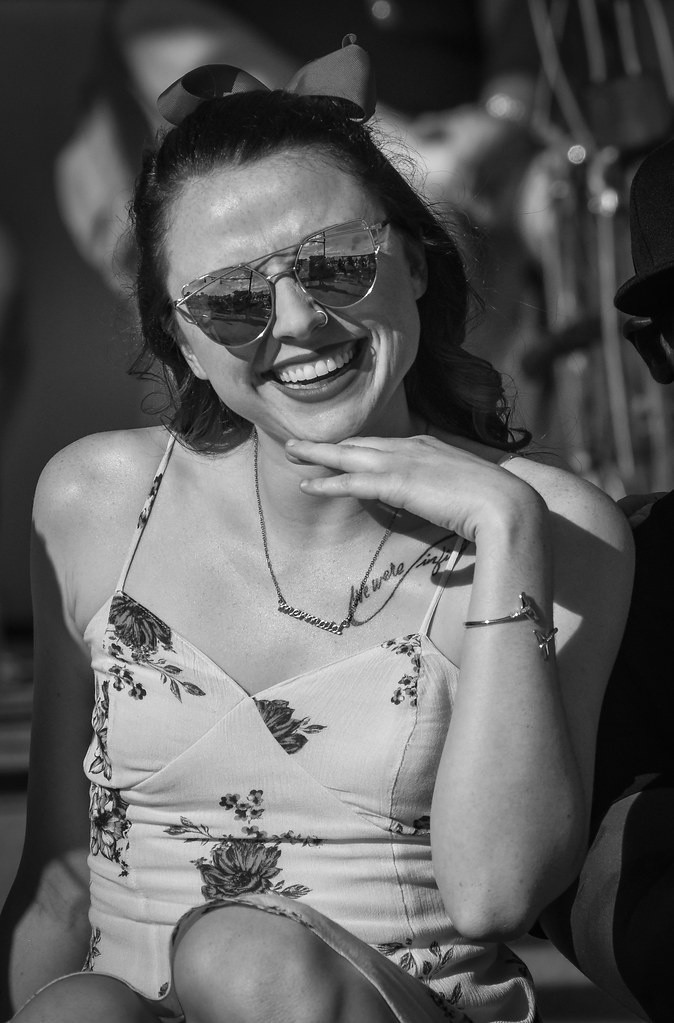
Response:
[[254, 418, 431, 637]]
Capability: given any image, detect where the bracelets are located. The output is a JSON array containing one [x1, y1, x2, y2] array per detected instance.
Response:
[[464, 591, 558, 658]]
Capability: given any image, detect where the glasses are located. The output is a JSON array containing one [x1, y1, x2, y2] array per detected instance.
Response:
[[169, 217, 392, 348]]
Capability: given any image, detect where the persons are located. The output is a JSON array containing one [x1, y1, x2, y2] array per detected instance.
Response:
[[0, 92, 636, 1023], [535, 139, 674, 1023]]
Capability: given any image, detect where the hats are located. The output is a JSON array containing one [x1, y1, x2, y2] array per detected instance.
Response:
[[613, 140, 674, 317]]
